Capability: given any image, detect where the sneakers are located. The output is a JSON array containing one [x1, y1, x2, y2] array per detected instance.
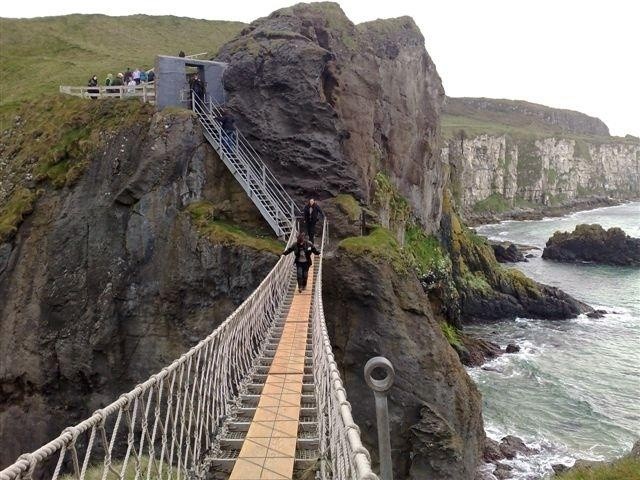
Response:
[[298, 287, 306, 293]]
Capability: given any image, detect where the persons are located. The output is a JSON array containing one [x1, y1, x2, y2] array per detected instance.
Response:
[[213, 110, 236, 153], [105, 67, 155, 100], [179, 50, 185, 58], [280, 233, 323, 293], [189, 73, 206, 111], [303, 198, 326, 244], [87, 75, 100, 100]]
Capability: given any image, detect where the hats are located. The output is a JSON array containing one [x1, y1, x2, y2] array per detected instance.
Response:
[[117, 72, 123, 78]]
